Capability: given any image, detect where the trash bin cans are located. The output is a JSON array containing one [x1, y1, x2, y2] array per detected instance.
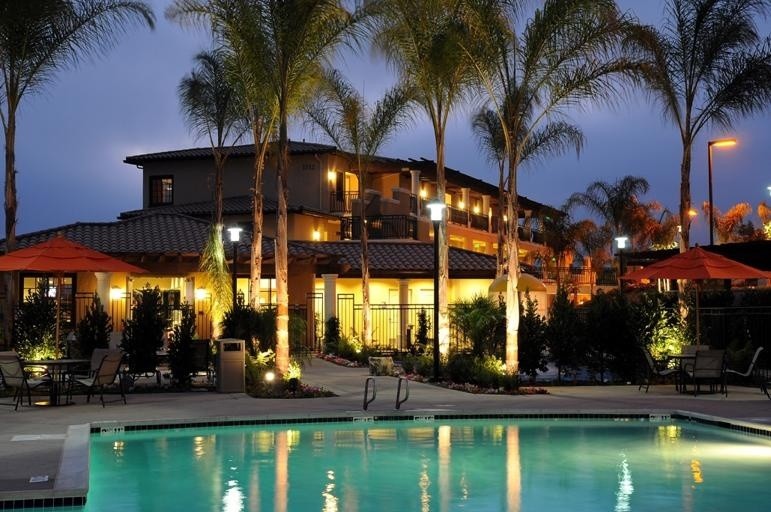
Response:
[[214, 338, 246, 393]]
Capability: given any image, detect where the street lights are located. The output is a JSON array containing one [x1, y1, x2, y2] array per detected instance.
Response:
[[613, 232, 630, 297], [707, 138, 739, 244], [425, 197, 448, 377], [227, 221, 244, 314]]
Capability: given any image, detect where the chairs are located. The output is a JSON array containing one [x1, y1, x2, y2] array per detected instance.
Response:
[[0, 347, 127, 411], [635, 344, 766, 397]]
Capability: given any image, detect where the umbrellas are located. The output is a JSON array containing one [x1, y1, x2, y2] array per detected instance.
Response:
[[0, 228, 151, 370], [613, 241, 771, 345]]
